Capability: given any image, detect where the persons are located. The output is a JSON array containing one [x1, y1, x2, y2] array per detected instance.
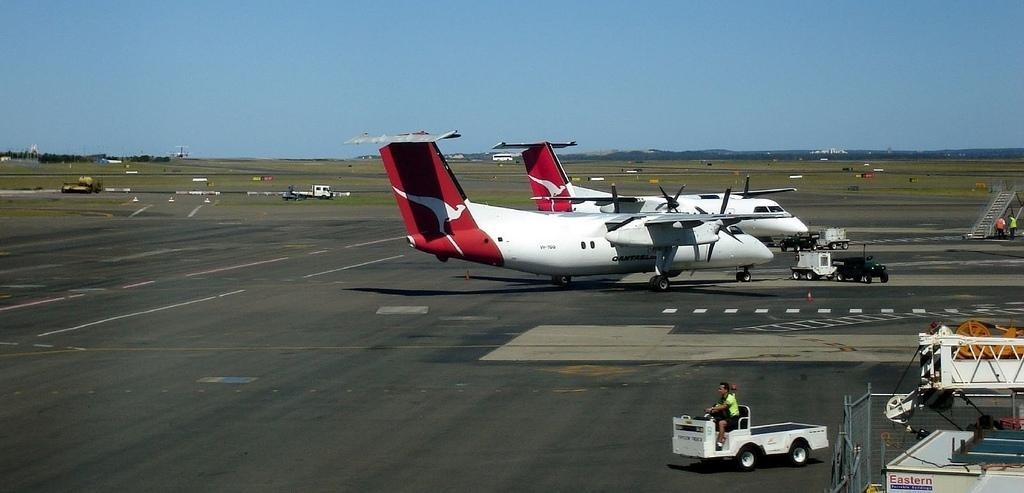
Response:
[[928, 319, 954, 337], [700, 382, 742, 445], [995, 212, 1005, 237], [1007, 214, 1019, 240]]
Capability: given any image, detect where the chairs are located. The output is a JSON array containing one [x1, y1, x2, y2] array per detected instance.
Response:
[[715, 404, 751, 436]]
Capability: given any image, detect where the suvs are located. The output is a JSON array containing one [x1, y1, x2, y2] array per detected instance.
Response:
[[780, 232, 820, 251], [833, 256, 889, 285]]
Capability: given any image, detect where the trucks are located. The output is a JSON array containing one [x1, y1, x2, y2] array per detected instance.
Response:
[[293, 185, 334, 199]]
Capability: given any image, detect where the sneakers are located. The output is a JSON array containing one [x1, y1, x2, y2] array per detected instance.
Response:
[[716, 446, 722, 451]]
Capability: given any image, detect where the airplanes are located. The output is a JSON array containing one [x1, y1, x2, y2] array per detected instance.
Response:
[[170, 145, 190, 158], [343, 129, 794, 295], [492, 138, 809, 246]]
